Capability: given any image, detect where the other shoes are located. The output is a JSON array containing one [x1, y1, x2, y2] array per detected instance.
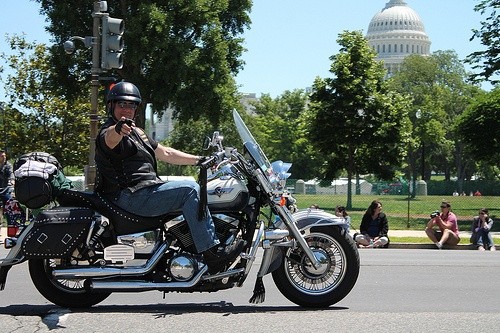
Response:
[[436, 242, 443, 249], [490, 246, 496, 251], [478, 246, 485, 251]]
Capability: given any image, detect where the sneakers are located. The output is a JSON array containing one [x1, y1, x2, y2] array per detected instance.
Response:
[[206, 239, 245, 275]]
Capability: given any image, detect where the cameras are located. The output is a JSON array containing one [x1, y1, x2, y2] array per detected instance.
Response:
[[430, 211, 440, 218]]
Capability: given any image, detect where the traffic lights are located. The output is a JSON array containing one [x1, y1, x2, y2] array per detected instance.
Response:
[[101, 14, 125, 70]]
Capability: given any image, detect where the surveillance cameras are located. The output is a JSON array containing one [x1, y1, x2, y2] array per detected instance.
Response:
[[64, 41, 74, 55]]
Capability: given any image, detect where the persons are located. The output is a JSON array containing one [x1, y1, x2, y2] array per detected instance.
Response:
[[453, 190, 481, 196], [335, 205, 350, 233], [425, 201, 460, 248], [470, 208, 496, 251], [353, 200, 389, 248], [311, 204, 319, 209], [95, 82, 245, 275], [0, 150, 15, 208]]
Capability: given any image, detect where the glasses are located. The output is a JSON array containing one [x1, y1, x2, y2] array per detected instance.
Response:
[[117, 102, 137, 110], [440, 206, 446, 209], [479, 212, 484, 215]]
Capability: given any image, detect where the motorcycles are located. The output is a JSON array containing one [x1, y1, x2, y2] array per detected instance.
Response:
[[0, 108, 362, 309]]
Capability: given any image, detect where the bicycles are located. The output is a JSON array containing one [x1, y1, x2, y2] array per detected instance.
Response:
[[0, 186, 26, 244]]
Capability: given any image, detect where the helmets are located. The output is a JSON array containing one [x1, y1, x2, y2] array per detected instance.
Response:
[[108, 81, 142, 106]]
